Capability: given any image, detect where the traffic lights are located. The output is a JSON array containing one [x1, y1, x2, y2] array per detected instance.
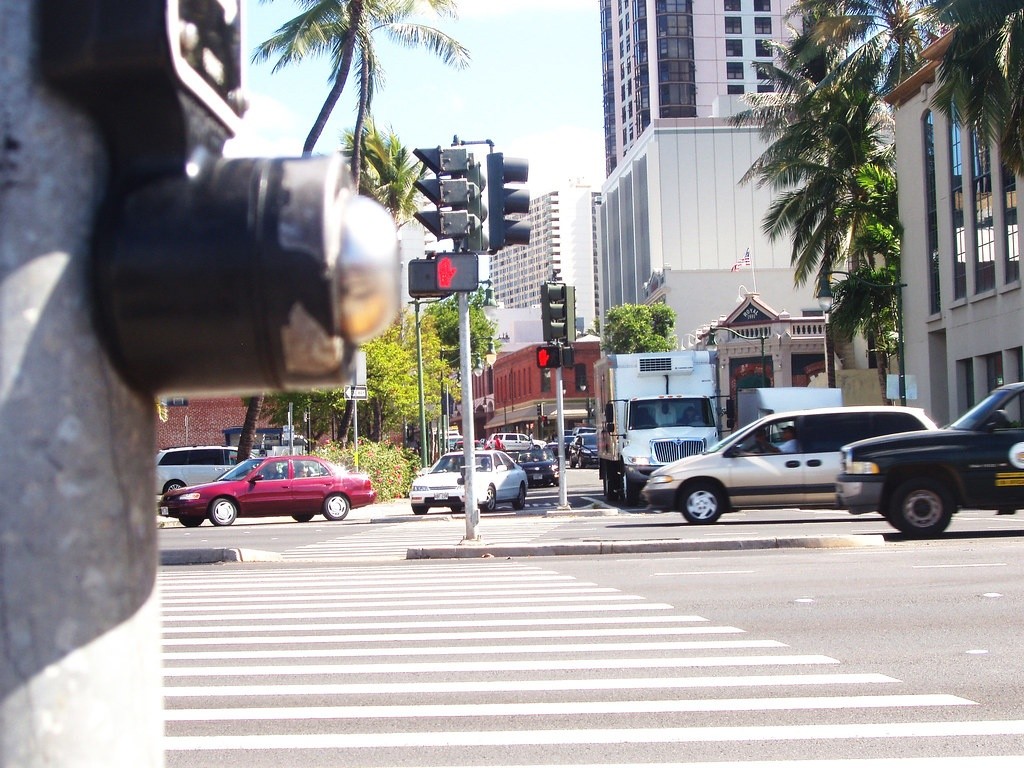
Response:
[[486, 152, 532, 251], [537, 403, 541, 415], [542, 415, 547, 421], [536, 346, 560, 369], [434, 251, 480, 292], [412, 144, 470, 241], [541, 283, 566, 342]]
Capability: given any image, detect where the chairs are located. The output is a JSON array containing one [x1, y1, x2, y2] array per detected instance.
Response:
[[476, 458, 491, 471], [636, 407, 656, 427]]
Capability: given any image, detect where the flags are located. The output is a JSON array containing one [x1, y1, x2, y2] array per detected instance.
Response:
[[731, 250, 750, 272]]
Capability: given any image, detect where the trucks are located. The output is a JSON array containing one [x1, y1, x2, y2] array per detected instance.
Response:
[[590, 348, 722, 509]]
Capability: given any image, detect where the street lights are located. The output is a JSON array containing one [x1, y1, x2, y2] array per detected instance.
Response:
[[704, 326, 767, 388], [407, 278, 501, 469], [439, 335, 498, 455], [814, 268, 908, 407]]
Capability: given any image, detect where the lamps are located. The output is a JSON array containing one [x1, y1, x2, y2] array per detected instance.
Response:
[[779, 363, 782, 367], [721, 365, 725, 369], [680, 323, 716, 351]]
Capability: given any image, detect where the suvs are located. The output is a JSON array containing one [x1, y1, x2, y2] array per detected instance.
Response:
[[832, 381, 1024, 541], [154, 444, 262, 496]]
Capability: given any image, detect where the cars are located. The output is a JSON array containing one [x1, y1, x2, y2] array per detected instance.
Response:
[[406, 449, 529, 516], [639, 404, 943, 526], [512, 448, 559, 489], [450, 425, 600, 469], [157, 455, 378, 528]]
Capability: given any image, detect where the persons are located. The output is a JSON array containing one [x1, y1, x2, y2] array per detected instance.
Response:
[[295, 462, 307, 477], [266, 461, 282, 480], [484, 435, 501, 450], [530, 432, 533, 439], [755, 426, 798, 453]]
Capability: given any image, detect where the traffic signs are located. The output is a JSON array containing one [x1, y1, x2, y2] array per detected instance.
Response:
[[343, 385, 369, 401]]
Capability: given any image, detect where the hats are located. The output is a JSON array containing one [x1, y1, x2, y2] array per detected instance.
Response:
[[781, 426, 794, 433]]
